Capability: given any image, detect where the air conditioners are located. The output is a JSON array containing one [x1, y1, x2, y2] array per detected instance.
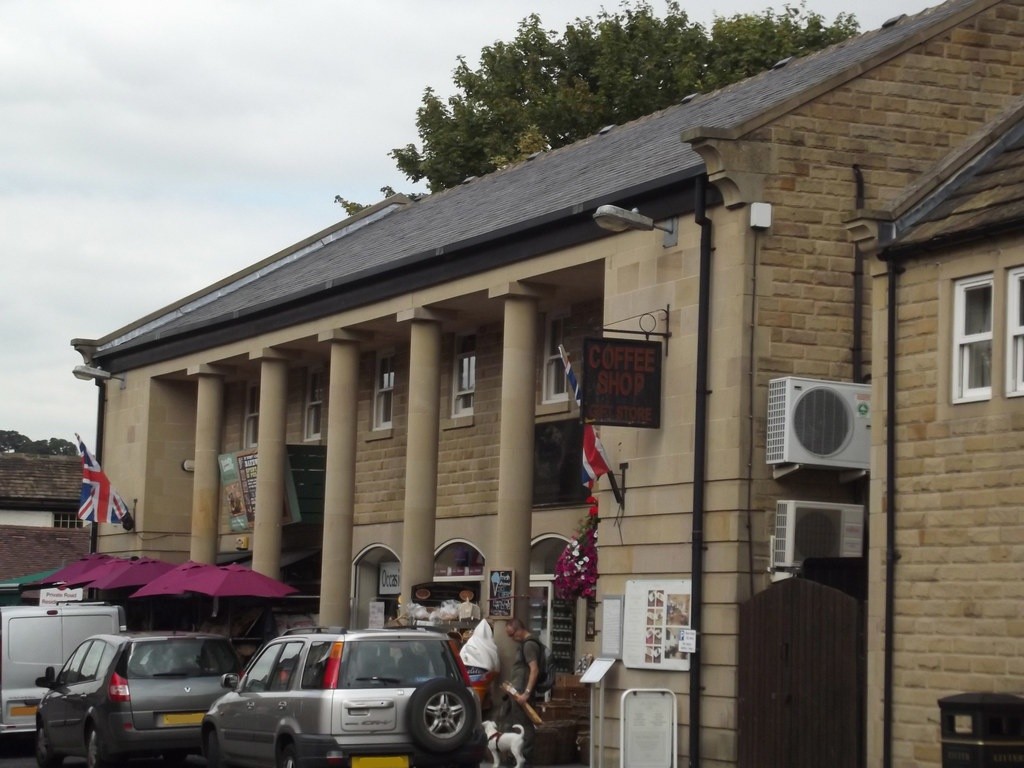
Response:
[[774, 501, 865, 568], [765, 376, 873, 472]]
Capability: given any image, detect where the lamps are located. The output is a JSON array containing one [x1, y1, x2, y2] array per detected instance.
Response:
[[592, 204, 679, 248], [72, 365, 127, 390]]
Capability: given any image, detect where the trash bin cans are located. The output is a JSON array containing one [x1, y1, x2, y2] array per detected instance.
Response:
[[937, 693, 1024, 768]]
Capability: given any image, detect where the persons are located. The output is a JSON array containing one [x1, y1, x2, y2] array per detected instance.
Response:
[[504, 620, 544, 768]]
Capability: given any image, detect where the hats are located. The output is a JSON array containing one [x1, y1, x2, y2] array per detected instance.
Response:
[[409, 641, 429, 659]]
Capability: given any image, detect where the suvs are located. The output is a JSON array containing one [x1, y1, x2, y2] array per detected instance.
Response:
[[201, 625, 482, 768]]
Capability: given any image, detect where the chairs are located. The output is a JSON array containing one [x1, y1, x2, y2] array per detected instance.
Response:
[[367, 654, 431, 680]]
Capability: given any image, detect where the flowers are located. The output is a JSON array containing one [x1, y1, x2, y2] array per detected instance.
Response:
[[555, 496, 599, 601]]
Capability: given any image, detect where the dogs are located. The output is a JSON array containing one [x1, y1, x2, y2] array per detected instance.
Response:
[[480, 720, 526, 768]]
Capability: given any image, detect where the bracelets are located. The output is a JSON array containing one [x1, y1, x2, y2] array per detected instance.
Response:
[[525, 689, 531, 693]]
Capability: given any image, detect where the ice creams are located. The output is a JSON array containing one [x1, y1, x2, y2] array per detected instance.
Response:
[[458, 618, 501, 707]]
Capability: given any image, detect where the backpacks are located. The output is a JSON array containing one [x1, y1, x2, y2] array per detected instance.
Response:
[[521, 639, 555, 694]]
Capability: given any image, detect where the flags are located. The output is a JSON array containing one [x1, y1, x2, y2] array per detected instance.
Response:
[[564, 362, 613, 488], [77, 440, 129, 523]]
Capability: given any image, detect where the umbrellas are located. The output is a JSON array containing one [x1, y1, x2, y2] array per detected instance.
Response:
[[62, 555, 130, 601], [181, 562, 300, 636], [40, 553, 112, 585], [83, 557, 178, 622], [129, 560, 219, 629]]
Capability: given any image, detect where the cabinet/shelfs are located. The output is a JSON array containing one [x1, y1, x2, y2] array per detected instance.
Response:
[[533, 604, 572, 660]]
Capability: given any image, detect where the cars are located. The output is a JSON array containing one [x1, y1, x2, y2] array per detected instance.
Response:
[[0, 602, 246, 768]]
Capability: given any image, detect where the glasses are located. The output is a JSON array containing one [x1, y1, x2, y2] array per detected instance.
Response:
[[509, 627, 518, 637]]
